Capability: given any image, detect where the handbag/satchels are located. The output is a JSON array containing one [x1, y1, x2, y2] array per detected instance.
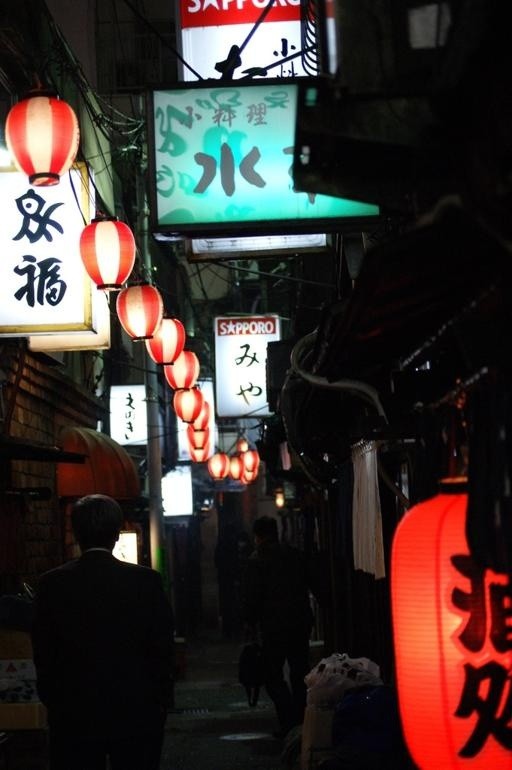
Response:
[[237, 642, 263, 706]]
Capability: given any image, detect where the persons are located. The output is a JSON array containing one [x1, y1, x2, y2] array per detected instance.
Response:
[[32, 491, 177, 770], [235, 513, 321, 728], [210, 521, 254, 641]]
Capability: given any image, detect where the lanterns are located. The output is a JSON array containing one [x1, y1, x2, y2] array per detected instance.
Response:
[[386, 476, 510, 768], [78, 214, 138, 292], [115, 281, 167, 342], [1, 95, 83, 190], [143, 314, 261, 487]]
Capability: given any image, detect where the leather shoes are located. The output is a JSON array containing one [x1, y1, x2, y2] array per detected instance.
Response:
[[273, 720, 298, 740]]
